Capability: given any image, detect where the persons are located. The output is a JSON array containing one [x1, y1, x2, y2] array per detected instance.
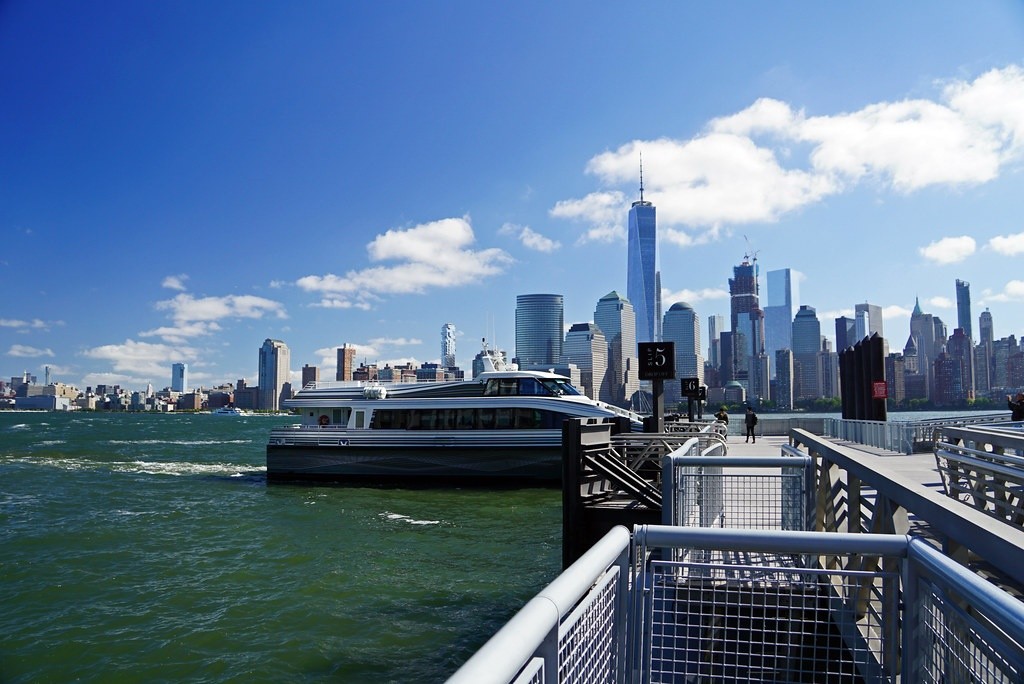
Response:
[[745, 407, 758, 444], [1007, 393, 1024, 456], [714, 407, 729, 440]]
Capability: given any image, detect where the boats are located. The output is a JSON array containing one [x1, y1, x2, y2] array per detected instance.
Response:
[[262, 371, 663, 477], [215, 406, 244, 416]]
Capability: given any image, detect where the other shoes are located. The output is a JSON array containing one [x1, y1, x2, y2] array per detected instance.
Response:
[[752, 442, 755, 443], [745, 440, 748, 443]]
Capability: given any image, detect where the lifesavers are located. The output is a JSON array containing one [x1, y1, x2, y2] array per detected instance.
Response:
[[318, 415, 329, 428]]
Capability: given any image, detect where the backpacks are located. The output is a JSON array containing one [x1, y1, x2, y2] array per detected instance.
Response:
[[752, 412, 758, 425]]
